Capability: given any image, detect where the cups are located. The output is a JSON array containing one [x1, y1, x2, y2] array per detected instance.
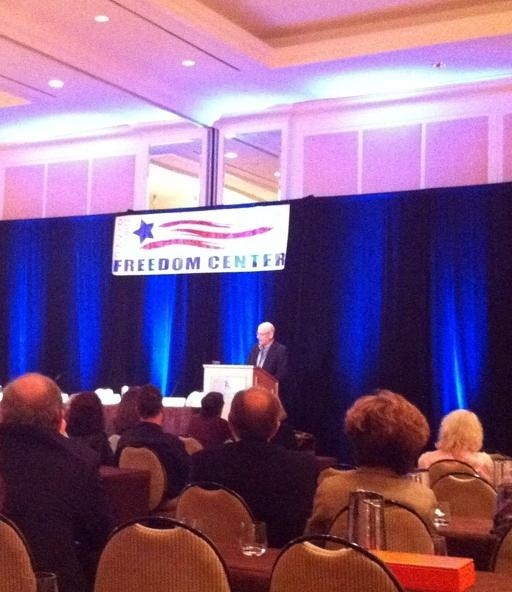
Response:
[[28, 572, 58, 591], [433, 502, 451, 528], [239, 520, 269, 557]]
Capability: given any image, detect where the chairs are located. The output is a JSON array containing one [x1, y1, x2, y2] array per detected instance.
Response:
[[1, 430, 511, 592]]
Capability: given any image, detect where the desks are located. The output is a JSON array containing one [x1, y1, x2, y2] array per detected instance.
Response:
[[59, 403, 203, 439]]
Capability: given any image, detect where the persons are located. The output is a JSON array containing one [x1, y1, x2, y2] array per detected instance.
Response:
[[249, 320, 289, 381]]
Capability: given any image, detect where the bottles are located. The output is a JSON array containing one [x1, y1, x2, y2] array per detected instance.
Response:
[[347, 490, 387, 550]]
